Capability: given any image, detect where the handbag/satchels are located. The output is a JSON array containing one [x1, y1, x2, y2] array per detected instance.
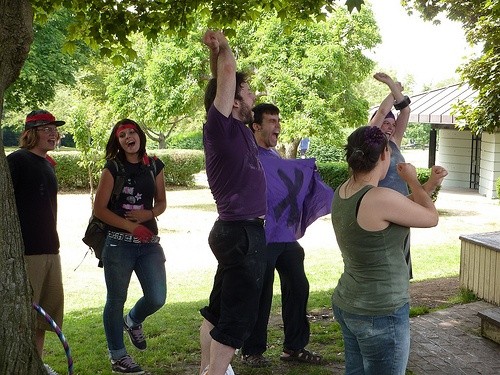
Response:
[[83, 156, 125, 258]]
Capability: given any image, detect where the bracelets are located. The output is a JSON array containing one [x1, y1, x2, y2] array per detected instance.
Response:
[[150, 209, 154, 221], [394, 95, 411, 110]]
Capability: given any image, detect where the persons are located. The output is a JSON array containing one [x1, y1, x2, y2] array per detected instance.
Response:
[[92, 120, 167, 375], [6, 110, 65, 375], [197, 28, 267, 375], [331, 126, 448, 375], [249, 103, 335, 364], [369, 73, 413, 280]]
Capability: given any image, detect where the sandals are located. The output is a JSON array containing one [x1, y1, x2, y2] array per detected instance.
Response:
[[280, 347, 321, 364], [241, 352, 269, 367]]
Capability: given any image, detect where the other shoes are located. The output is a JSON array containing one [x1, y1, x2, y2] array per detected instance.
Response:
[[43, 363, 58, 375]]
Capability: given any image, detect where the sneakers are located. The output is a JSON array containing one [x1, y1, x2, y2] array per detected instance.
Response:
[[111, 355, 145, 375], [122, 315, 147, 351]]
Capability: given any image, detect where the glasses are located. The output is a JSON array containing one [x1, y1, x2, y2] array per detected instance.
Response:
[[36, 127, 58, 136]]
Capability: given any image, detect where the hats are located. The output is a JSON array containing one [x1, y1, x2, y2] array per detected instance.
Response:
[[25, 112, 66, 131]]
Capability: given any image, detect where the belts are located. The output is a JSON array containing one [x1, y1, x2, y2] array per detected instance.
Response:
[[108, 231, 161, 244], [218, 216, 267, 226]]
